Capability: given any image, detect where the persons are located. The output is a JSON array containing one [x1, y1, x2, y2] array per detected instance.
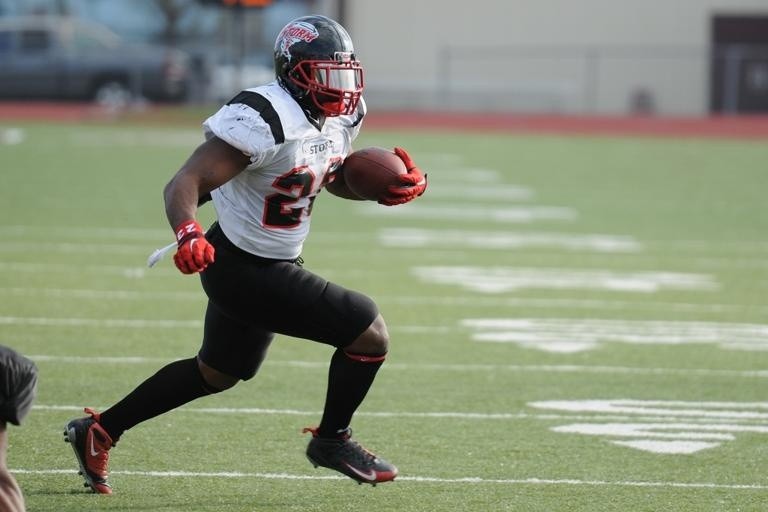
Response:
[[58, 12, 431, 496], [0, 341, 45, 511]]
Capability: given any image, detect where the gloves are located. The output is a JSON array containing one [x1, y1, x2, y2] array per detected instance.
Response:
[[378, 147, 429, 206], [173, 220, 216, 275]]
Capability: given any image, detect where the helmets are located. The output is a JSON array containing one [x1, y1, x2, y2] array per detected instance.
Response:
[[274, 14, 365, 117]]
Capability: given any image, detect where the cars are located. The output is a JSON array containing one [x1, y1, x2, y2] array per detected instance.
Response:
[[0, 14, 197, 108]]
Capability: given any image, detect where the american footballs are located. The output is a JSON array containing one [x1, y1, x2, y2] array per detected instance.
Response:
[[344, 147, 407, 200]]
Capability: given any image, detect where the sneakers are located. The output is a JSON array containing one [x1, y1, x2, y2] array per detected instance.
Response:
[[63, 407, 115, 494], [302, 425, 398, 487]]
